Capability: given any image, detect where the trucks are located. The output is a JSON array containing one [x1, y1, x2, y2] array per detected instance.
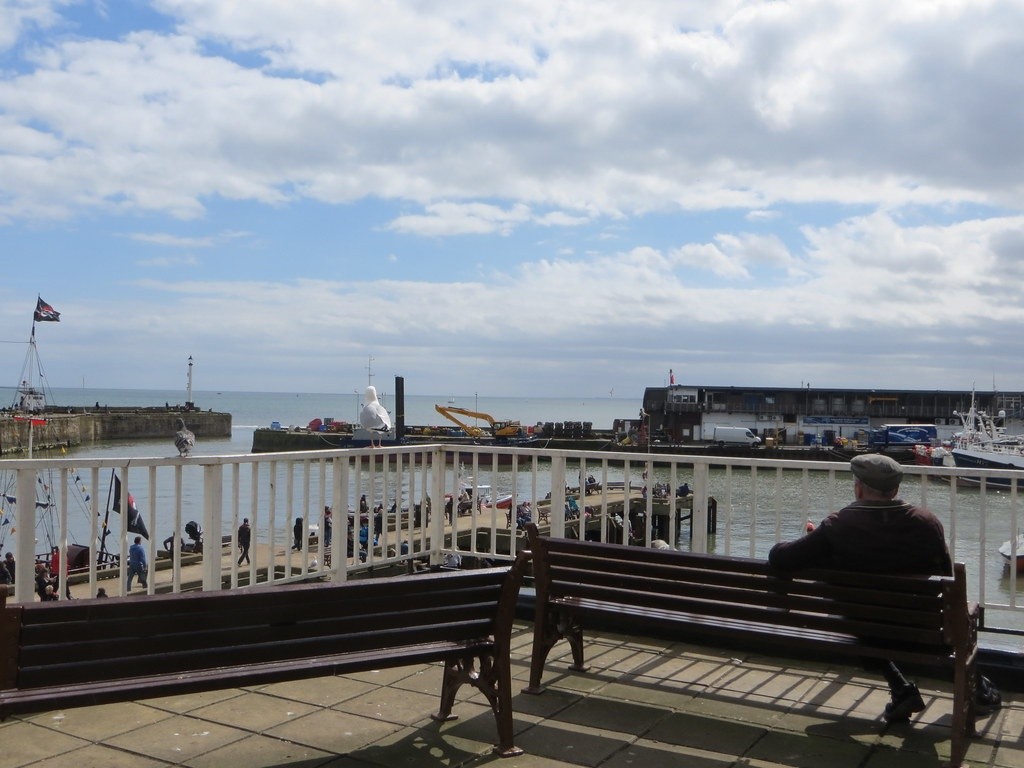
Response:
[[868, 424, 937, 443]]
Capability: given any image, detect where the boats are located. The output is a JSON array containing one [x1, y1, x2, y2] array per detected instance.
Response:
[[999, 528, 1024, 569], [0, 293, 131, 583], [912, 377, 1024, 492]]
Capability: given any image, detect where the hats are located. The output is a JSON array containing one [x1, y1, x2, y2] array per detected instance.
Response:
[[849, 453, 903, 490]]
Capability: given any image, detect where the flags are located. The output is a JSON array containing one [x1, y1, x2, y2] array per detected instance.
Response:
[[34, 296, 61, 322], [112, 475, 150, 541]]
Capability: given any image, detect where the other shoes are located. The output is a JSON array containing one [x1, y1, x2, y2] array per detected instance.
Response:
[[976, 688, 1001, 714], [238, 563, 242, 567], [885, 682, 926, 721]]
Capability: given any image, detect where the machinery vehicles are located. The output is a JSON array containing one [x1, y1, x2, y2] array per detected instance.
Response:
[[434, 403, 532, 444]]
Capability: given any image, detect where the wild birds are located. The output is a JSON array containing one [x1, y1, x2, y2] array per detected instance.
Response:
[[172, 415, 196, 457], [361, 385, 392, 448]]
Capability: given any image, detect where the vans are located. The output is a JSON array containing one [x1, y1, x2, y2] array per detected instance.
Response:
[[714, 427, 762, 448]]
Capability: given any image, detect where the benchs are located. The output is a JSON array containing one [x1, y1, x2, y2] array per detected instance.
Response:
[[586, 481, 600, 494], [0, 548, 534, 757], [524, 522, 984, 767], [505, 508, 548, 529], [445, 498, 483, 519], [600, 480, 631, 494], [565, 510, 579, 521]]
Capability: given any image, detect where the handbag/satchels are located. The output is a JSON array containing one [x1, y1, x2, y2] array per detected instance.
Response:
[[137, 569, 147, 583]]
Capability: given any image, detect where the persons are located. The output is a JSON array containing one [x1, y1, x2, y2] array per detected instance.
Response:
[[462, 492, 469, 501], [164, 531, 185, 580], [127, 536, 148, 592], [585, 475, 595, 494], [97, 588, 108, 598], [237, 518, 251, 567], [509, 502, 532, 531], [324, 493, 383, 556], [401, 540, 408, 555], [768, 454, 1001, 721], [447, 497, 453, 526], [0, 552, 15, 596], [292, 518, 303, 551], [642, 482, 689, 498], [35, 547, 71, 602]]
